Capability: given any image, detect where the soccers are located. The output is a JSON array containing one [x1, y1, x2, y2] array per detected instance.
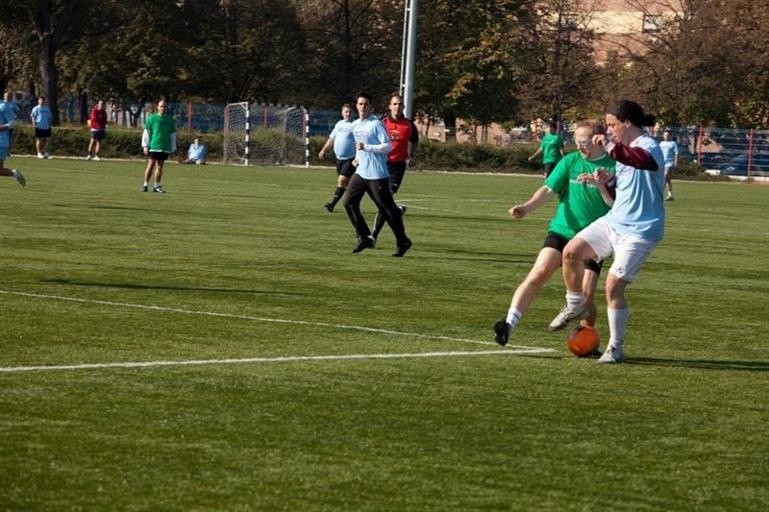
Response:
[[195, 158, 203, 165], [569, 326, 599, 357]]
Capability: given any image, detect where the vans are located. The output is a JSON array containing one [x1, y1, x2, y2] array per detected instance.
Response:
[[277, 109, 383, 136], [162, 101, 281, 133]]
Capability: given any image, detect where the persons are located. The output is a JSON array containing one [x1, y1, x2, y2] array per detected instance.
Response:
[[528, 122, 564, 180], [368, 92, 419, 249], [0, 91, 20, 157], [318, 103, 356, 212], [85, 100, 108, 162], [1, 99, 26, 186], [659, 130, 679, 201], [342, 90, 412, 257], [141, 96, 177, 193], [548, 100, 665, 364], [30, 96, 52, 159], [178, 137, 207, 166], [493, 119, 617, 346]]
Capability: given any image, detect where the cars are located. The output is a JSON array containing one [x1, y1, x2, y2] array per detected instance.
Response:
[[690, 151, 735, 169], [713, 153, 769, 176], [691, 127, 769, 151], [511, 123, 691, 146]]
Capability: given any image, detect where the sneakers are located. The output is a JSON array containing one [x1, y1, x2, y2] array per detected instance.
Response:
[[154, 186, 165, 193], [13, 169, 25, 186], [38, 153, 98, 161], [326, 201, 412, 257], [141, 185, 147, 191], [494, 307, 623, 363]]
